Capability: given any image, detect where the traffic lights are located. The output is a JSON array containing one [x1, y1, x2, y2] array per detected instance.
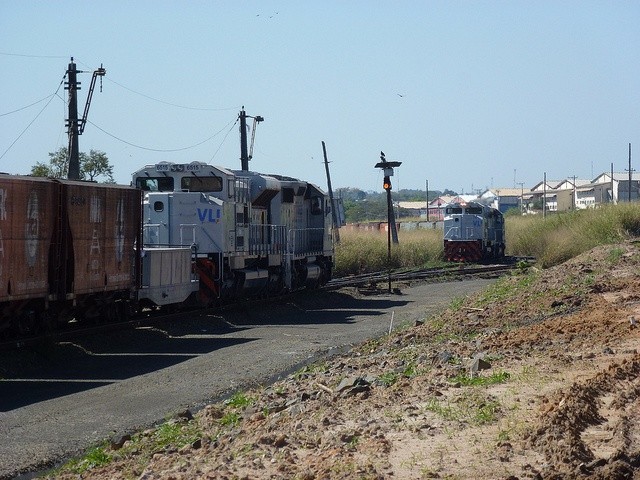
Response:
[[383, 177, 392, 190]]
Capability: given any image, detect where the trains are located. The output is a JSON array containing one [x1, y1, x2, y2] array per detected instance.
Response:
[[0, 160, 335, 324], [339, 201, 505, 263]]
